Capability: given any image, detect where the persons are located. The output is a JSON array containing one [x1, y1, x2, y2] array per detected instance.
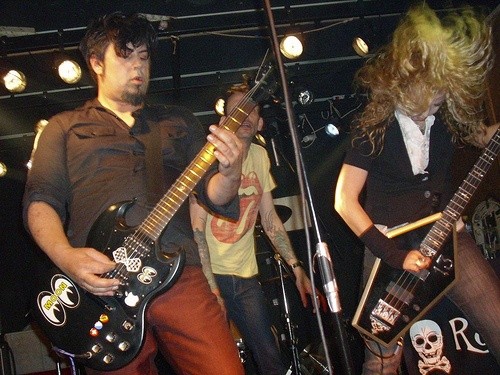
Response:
[[189, 89, 327, 375], [22, 12, 244, 375], [334, 0, 500, 375]]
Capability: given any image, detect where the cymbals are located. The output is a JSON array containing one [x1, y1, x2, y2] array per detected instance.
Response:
[[255, 204, 292, 227]]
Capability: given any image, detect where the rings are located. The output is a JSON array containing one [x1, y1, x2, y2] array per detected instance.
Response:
[[416, 257, 422, 266], [316, 296, 319, 299]]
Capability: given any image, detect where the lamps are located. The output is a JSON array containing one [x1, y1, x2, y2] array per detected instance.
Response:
[[353, 23, 380, 57], [0, 59, 29, 95], [32, 113, 49, 135], [268, 78, 341, 168], [214, 94, 224, 118], [50, 53, 83, 84], [279, 24, 310, 59]]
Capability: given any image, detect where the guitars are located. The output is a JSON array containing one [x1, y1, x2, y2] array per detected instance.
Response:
[[349, 126, 500, 351], [30, 46, 292, 372]]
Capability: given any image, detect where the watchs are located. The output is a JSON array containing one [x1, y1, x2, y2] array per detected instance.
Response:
[[291, 260, 304, 270]]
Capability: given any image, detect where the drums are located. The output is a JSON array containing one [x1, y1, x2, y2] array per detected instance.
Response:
[[225, 316, 253, 366], [252, 250, 293, 282]]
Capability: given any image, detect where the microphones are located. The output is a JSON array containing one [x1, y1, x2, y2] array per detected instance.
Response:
[[271, 139, 279, 166]]
